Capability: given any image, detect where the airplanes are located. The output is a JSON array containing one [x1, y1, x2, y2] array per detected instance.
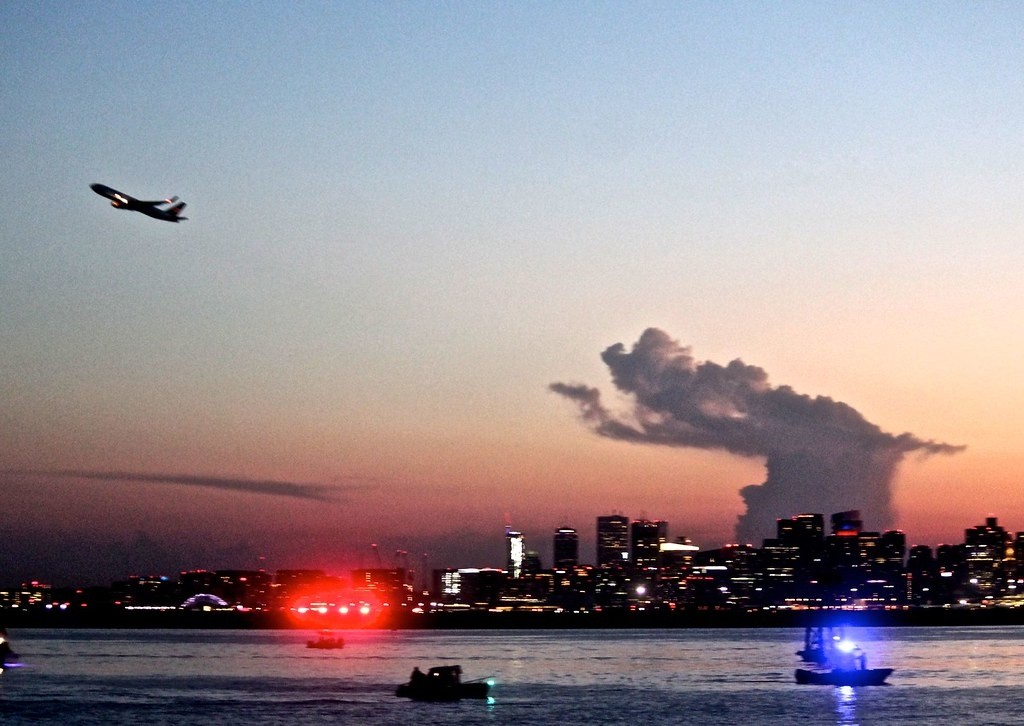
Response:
[[89, 183, 188, 224]]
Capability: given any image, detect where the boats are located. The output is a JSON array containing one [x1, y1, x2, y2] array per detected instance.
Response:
[[794, 616, 894, 686], [396, 665, 495, 699], [306, 629, 346, 648]]
